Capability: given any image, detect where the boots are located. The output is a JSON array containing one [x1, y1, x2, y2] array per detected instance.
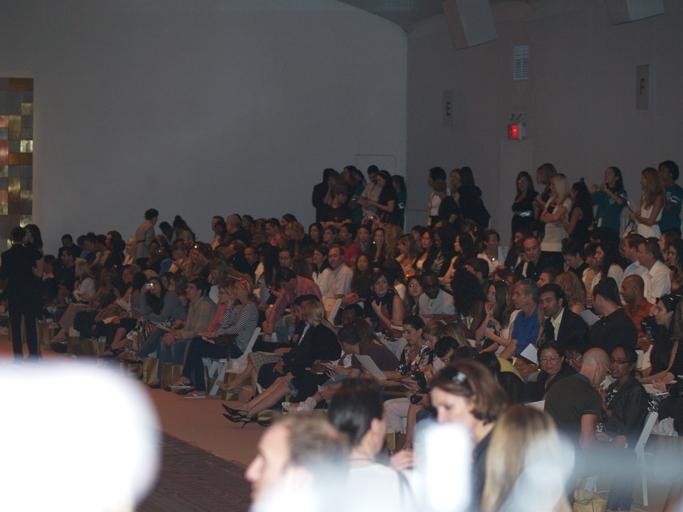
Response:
[[281, 396, 317, 415]]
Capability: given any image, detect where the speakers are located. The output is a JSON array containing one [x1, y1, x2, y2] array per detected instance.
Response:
[[606, 0, 665, 25], [443, 0, 498, 49]]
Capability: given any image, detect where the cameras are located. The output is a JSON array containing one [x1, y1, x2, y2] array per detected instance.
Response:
[[146, 283, 154, 289], [410, 372, 430, 404]]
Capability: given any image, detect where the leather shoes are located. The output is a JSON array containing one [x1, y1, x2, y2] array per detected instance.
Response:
[[145, 379, 172, 391], [10, 352, 42, 367], [214, 379, 257, 401], [120, 354, 148, 365], [76, 337, 93, 354], [48, 330, 68, 348], [386, 432, 397, 457]]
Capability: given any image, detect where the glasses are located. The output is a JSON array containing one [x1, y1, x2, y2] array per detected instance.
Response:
[[608, 357, 633, 365]]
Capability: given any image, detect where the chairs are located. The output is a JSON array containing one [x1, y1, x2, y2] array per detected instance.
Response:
[[202, 327, 263, 397], [584, 410, 658, 506], [322, 296, 342, 324]]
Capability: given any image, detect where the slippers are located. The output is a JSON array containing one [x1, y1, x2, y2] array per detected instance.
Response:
[[182, 391, 207, 400], [167, 380, 192, 390]]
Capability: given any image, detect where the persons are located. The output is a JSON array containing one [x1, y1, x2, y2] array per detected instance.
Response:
[[21, 224, 45, 360], [389, 359, 511, 512], [0, 227, 44, 365], [44, 160, 682, 454], [544, 346, 639, 512], [244, 412, 350, 512], [480, 404, 572, 512], [326, 384, 430, 512]]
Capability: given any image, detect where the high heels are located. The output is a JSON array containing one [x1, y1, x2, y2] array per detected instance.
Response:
[[222, 412, 252, 429], [97, 342, 124, 361], [221, 402, 250, 416]]
